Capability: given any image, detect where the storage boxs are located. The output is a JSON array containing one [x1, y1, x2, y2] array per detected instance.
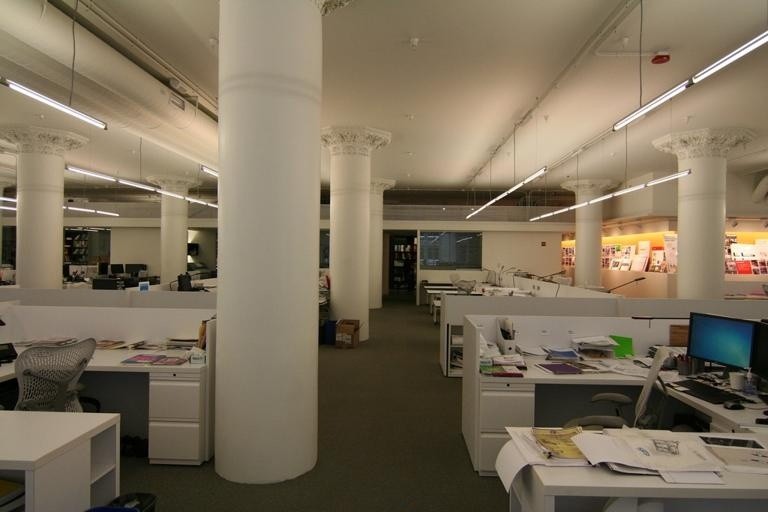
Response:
[[336, 320, 360, 349]]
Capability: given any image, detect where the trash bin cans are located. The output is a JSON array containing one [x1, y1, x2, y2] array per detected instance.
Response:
[[109, 492, 159, 512], [324, 320, 336, 345]]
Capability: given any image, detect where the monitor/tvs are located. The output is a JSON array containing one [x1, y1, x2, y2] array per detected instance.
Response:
[[96, 261, 109, 276], [687, 312, 755, 380]]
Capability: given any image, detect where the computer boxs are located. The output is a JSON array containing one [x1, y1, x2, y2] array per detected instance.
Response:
[[125, 263, 146, 277], [92, 277, 126, 290]]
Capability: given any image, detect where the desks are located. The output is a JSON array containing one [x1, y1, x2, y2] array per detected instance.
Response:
[[0, 308, 216, 385], [58, 271, 218, 306], [462, 315, 768, 511], [419, 274, 626, 377]]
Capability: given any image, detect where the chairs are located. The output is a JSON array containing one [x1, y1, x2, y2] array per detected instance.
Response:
[[565, 345, 670, 430], [0, 337, 100, 412]]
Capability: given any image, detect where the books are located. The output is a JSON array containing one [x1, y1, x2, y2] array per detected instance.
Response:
[[494, 427, 768, 494], [479, 335, 619, 378], [63, 231, 87, 263], [0, 479, 25, 511], [12, 339, 206, 365]]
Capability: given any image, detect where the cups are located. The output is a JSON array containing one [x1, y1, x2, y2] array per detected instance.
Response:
[[730, 372, 744, 390], [677, 361, 691, 375]]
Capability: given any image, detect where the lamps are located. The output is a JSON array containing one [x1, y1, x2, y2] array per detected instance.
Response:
[[466, 166, 546, 219], [66, 164, 218, 217], [613, 78, 694, 131], [693, 30, 767, 82], [609, 278, 646, 292], [1, 76, 109, 131], [529, 168, 691, 222]]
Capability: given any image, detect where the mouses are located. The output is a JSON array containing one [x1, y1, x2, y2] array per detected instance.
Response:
[[724, 401, 745, 410]]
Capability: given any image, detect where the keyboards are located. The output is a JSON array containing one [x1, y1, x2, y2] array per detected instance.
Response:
[[673, 380, 745, 404]]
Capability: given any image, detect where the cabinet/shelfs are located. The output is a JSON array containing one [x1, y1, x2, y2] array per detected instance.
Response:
[[63, 228, 87, 270], [88, 229, 111, 274], [150, 375, 204, 468], [1, 410, 121, 511], [480, 384, 535, 474], [447, 323, 463, 377]]
